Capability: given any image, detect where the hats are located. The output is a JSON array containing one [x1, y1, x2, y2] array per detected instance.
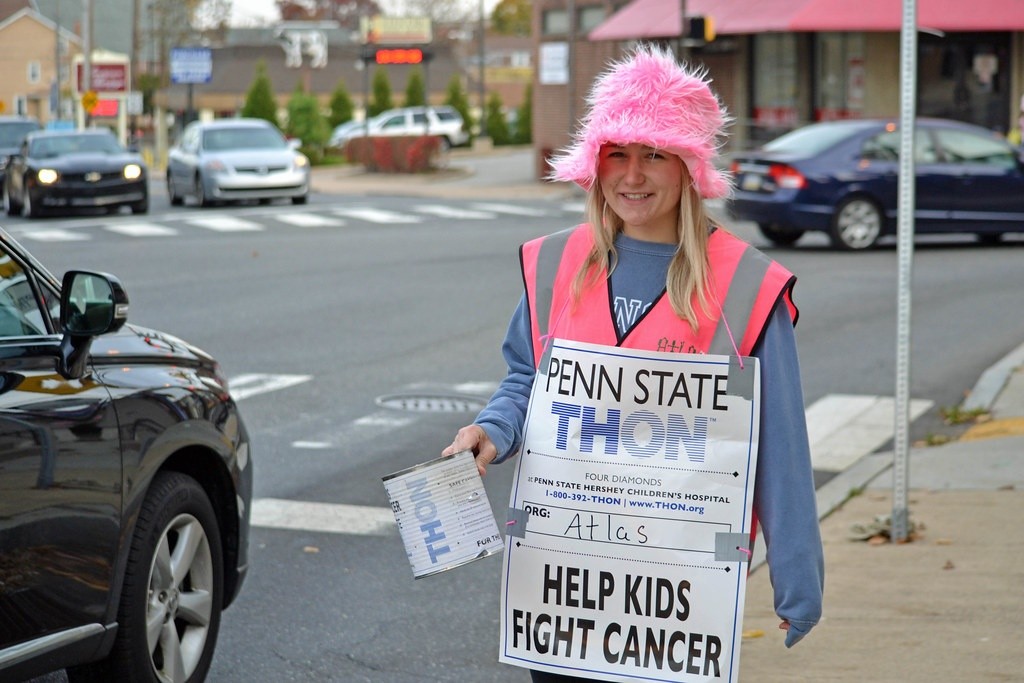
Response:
[[545, 45, 736, 199]]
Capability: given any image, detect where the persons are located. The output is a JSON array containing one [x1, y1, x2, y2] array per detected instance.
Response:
[[440, 39, 824, 683], [1007, 110, 1024, 155]]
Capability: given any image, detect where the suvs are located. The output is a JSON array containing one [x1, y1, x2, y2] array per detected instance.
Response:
[[0, 116, 43, 201], [327, 104, 470, 152], [0, 224, 255, 683]]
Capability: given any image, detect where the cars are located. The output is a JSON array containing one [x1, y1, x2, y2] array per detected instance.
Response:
[[164, 117, 311, 208], [0, 127, 149, 218], [724, 117, 1024, 249]]
